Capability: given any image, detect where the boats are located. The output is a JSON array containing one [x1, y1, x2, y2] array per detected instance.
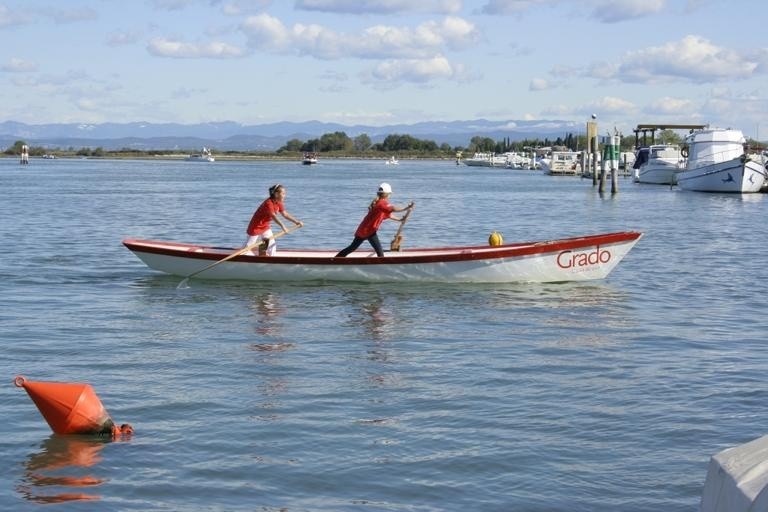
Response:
[[300, 157, 318, 164], [122, 229, 642, 283], [184, 152, 216, 162], [383, 156, 399, 166], [40, 154, 57, 159]]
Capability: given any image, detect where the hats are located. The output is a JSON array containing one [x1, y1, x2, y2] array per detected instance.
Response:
[[378, 183, 393, 194]]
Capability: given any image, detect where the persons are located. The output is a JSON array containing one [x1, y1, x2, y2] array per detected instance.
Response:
[[335, 183, 415, 257], [303, 151, 320, 160], [244, 183, 302, 255]]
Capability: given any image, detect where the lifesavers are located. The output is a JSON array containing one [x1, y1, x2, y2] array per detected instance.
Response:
[[681, 147, 689, 158]]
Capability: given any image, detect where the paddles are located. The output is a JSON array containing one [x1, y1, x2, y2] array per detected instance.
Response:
[[187, 221, 301, 278]]
